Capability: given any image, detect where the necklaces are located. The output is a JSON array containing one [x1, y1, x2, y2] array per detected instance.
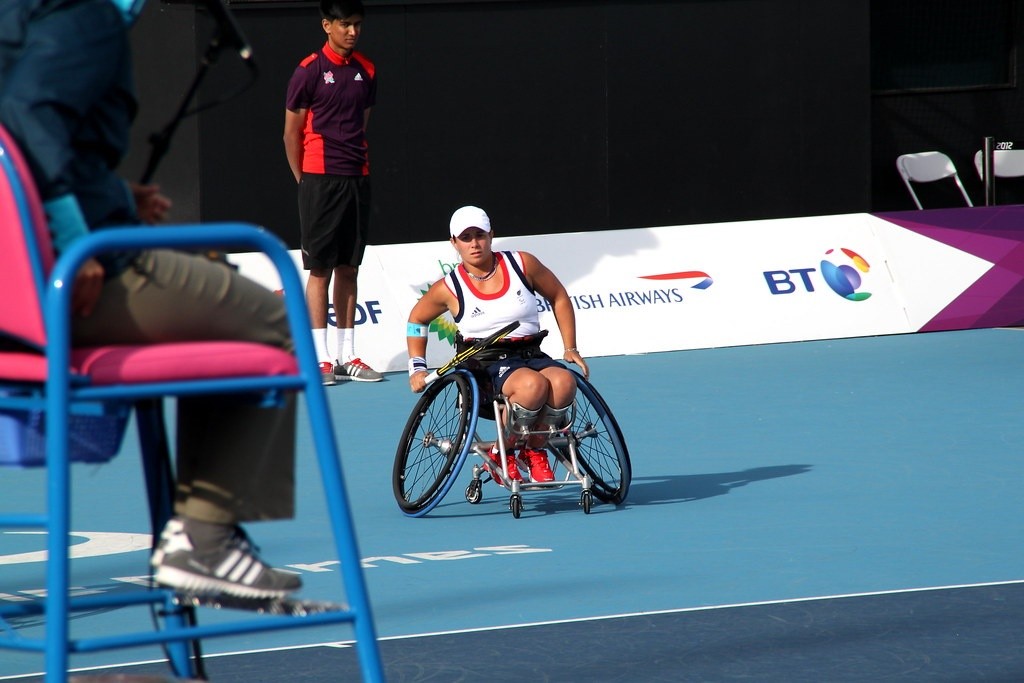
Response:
[[460, 251, 498, 282]]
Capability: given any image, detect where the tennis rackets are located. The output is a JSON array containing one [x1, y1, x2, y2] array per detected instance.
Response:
[[424, 320, 521, 385]]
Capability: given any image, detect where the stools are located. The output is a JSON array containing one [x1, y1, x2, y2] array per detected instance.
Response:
[[0, 123, 390, 683]]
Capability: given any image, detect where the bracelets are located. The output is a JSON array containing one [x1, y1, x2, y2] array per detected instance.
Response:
[[564, 347, 578, 353], [407, 357, 427, 377]]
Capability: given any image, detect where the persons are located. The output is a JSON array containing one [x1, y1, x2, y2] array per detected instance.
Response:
[[0, 0, 304, 601], [282, 1, 384, 386], [405, 206, 590, 490]]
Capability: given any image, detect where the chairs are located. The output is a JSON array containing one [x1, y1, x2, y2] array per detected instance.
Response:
[[897, 151, 977, 211]]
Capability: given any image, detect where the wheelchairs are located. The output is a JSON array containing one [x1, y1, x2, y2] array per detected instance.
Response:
[[392, 329, 632, 521]]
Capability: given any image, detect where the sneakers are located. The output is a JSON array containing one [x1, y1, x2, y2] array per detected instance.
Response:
[[319, 361, 337, 385], [514, 443, 555, 483], [483, 445, 524, 488], [151, 515, 303, 600], [333, 357, 384, 382]]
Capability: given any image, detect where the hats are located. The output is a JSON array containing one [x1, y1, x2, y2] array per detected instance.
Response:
[[450, 206, 491, 238]]
